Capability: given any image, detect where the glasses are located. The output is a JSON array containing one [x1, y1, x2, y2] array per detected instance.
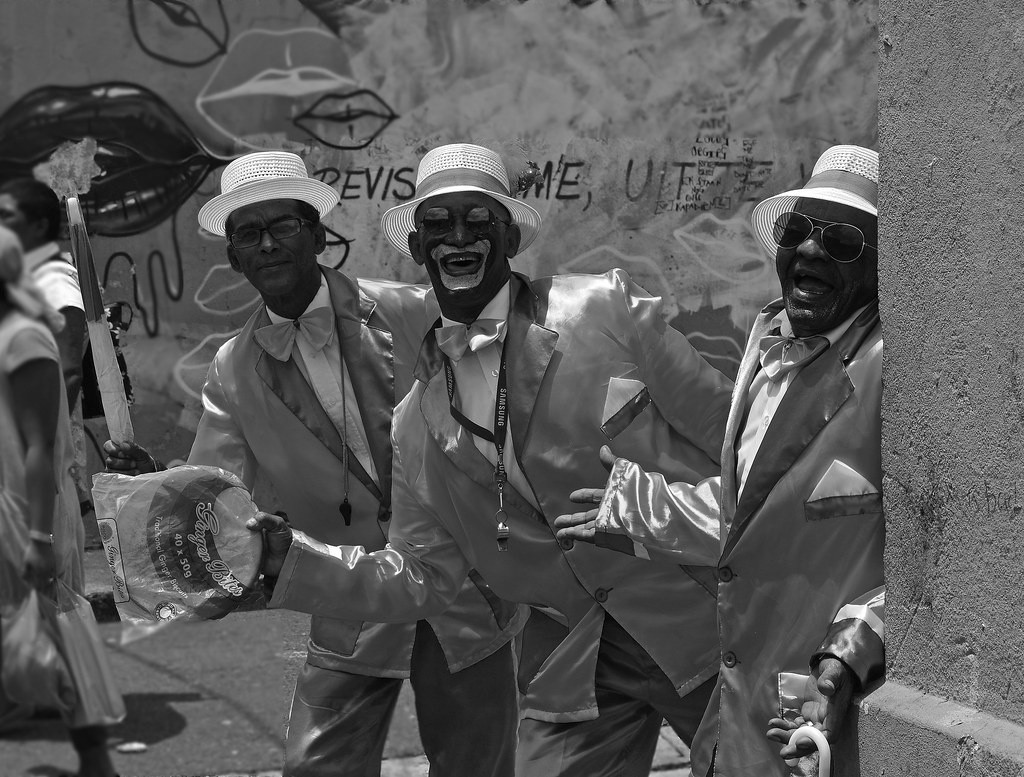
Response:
[[772, 212, 878, 264], [229, 217, 314, 250], [416, 206, 508, 236]]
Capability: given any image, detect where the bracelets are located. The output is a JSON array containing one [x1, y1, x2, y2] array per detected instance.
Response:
[[29, 530, 56, 544]]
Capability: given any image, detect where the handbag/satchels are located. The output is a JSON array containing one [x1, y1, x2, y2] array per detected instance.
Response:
[[0, 586, 76, 722], [54, 577, 127, 728]]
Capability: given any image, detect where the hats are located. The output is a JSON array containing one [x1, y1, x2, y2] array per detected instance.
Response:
[[381, 142, 542, 261], [751, 144, 879, 264], [198, 150, 341, 238]]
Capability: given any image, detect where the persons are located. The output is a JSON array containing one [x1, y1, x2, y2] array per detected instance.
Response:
[[243, 142, 735, 777], [553, 142, 885, 777], [0, 177, 92, 514], [0, 229, 122, 777], [102, 151, 532, 777]]
